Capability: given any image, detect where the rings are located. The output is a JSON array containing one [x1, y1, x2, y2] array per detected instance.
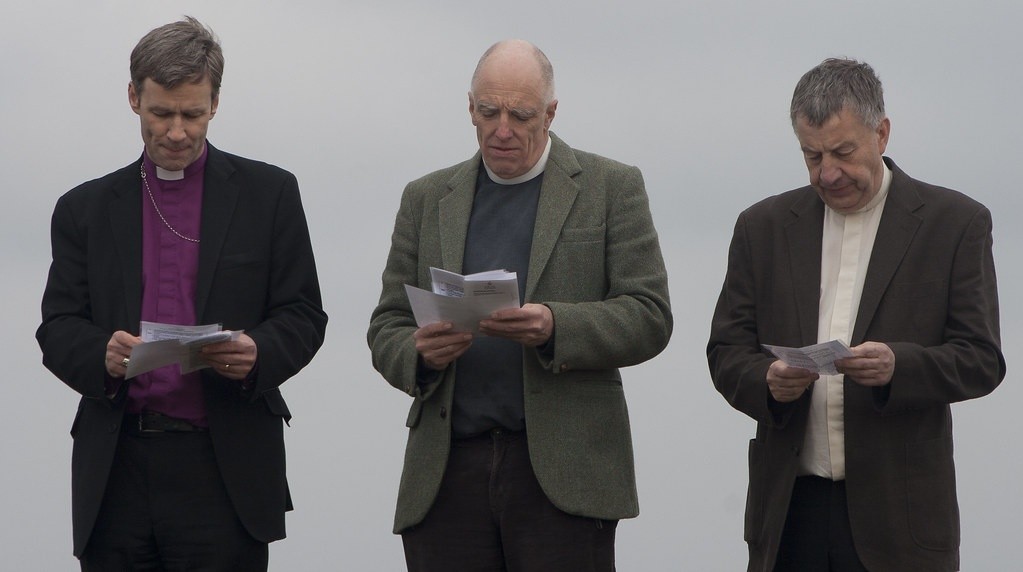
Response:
[[122, 356, 130, 366], [225, 364, 230, 371]]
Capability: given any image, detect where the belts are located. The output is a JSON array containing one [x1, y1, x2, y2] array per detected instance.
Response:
[[122, 410, 209, 432]]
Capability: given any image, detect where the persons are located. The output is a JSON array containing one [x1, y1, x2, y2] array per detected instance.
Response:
[[707, 57, 1007, 572], [35, 14, 328, 572], [367, 39, 674, 572]]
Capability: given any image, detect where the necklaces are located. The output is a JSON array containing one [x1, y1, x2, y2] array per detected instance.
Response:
[[141, 162, 200, 243]]
